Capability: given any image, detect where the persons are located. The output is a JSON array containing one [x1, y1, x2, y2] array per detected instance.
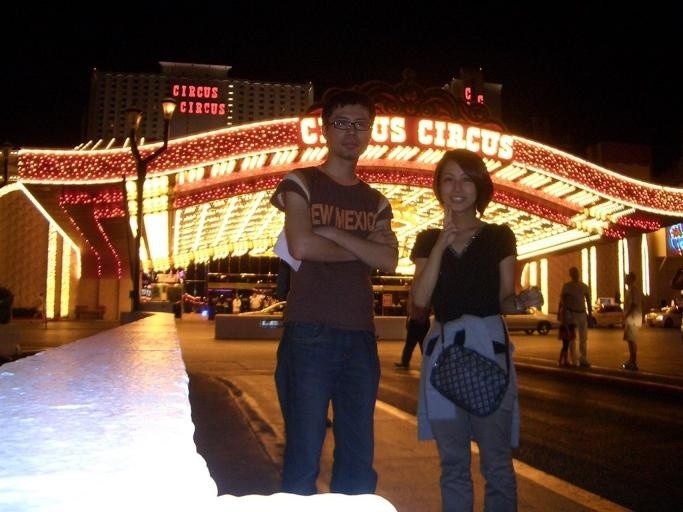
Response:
[[394, 298, 433, 370], [557, 267, 593, 367], [28, 292, 45, 324], [659, 299, 666, 310], [268, 85, 400, 496], [619, 272, 643, 371], [185, 288, 278, 322], [408, 148, 539, 512]]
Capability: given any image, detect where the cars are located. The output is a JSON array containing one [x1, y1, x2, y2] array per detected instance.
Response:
[[644, 305, 681, 328], [503, 306, 561, 335], [587, 304, 623, 329]]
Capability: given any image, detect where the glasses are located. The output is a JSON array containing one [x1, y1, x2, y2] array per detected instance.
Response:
[[325, 119, 373, 131]]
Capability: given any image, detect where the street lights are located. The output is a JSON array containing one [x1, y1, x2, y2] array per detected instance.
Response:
[[124, 96, 177, 312]]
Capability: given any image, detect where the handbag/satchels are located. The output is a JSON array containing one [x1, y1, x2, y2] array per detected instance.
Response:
[[430, 343, 509, 417]]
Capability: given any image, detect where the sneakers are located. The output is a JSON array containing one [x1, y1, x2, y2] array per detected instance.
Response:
[[618, 360, 639, 370]]
